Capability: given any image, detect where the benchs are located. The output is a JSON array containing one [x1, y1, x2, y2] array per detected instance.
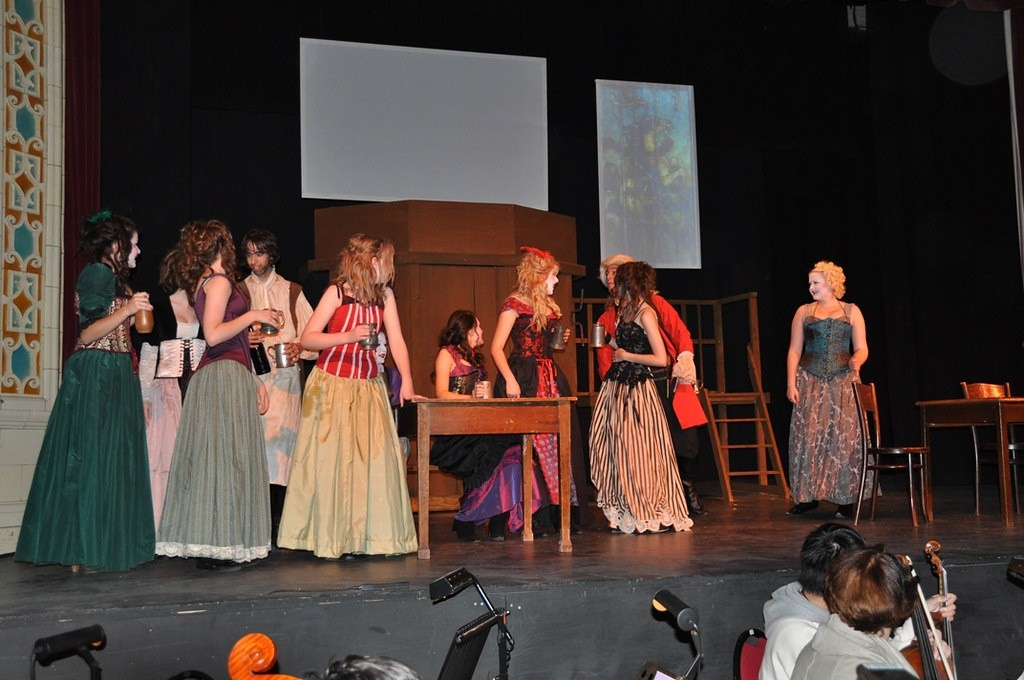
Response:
[[404, 466, 488, 540]]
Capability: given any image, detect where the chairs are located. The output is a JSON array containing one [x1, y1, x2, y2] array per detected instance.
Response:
[[959, 381, 1024, 516], [733, 628, 768, 680], [852, 381, 930, 527]]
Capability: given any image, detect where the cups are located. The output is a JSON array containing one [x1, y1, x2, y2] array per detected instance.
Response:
[[479, 380, 492, 399], [262, 308, 285, 334], [267, 342, 294, 368], [548, 326, 566, 350], [590, 324, 605, 347], [359, 322, 378, 350]]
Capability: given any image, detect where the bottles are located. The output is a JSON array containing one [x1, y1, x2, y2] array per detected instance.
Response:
[[135, 309, 154, 333], [248, 324, 271, 376]]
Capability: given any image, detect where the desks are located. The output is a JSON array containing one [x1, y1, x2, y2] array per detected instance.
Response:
[[916, 398, 1023, 528], [393, 397, 578, 561]]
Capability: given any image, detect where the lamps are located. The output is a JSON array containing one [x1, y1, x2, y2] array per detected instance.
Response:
[[30, 624, 108, 680], [652, 589, 705, 680], [430, 566, 516, 680]]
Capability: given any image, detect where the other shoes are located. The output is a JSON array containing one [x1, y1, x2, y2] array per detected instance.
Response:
[[785, 500, 819, 515], [196, 557, 241, 572], [452, 520, 482, 543], [488, 516, 506, 541], [835, 504, 854, 519], [240, 559, 260, 567]]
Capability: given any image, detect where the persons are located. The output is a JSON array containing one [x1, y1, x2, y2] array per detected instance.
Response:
[[274, 233, 429, 559], [138, 218, 321, 572], [433, 309, 541, 544], [12, 211, 154, 576], [490, 246, 709, 531], [785, 259, 881, 518], [758, 522, 957, 680]]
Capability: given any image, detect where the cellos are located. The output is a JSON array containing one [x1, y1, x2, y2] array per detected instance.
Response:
[[924, 540, 954, 663], [895, 551, 953, 680], [227, 632, 303, 680]]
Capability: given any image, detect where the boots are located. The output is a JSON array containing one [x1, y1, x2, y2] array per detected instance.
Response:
[[686, 486, 708, 515]]
